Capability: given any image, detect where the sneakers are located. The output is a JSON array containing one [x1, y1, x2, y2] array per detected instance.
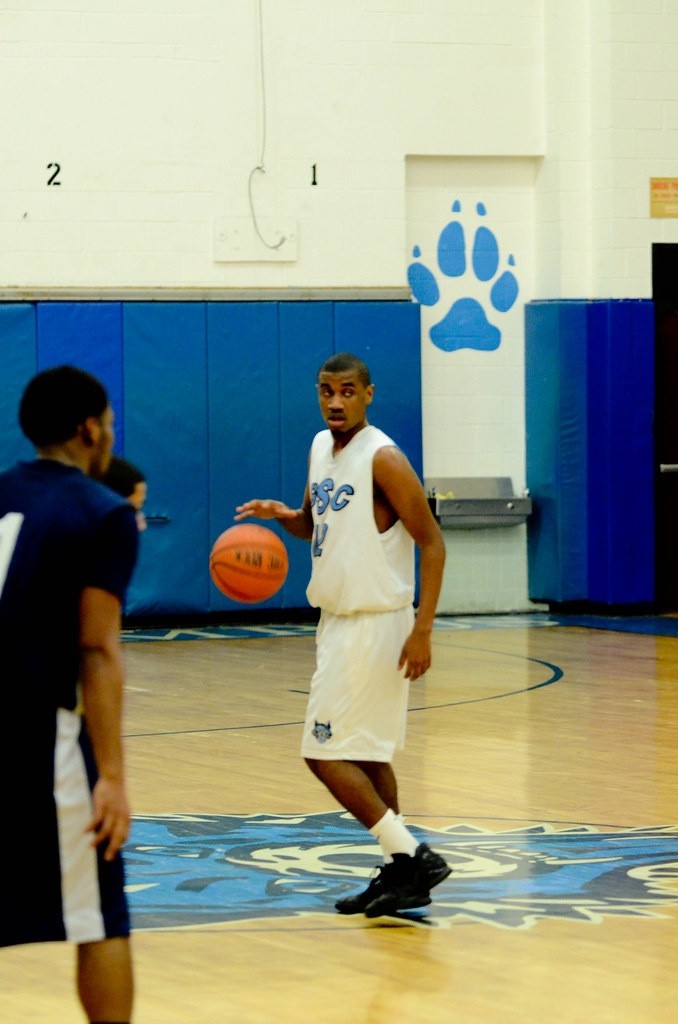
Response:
[[335, 842, 452, 919]]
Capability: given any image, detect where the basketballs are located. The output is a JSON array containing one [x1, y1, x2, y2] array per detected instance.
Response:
[[207, 524, 288, 603]]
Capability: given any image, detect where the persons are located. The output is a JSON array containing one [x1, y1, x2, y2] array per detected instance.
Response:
[[234, 352, 454, 917], [0, 364, 152, 1024]]
